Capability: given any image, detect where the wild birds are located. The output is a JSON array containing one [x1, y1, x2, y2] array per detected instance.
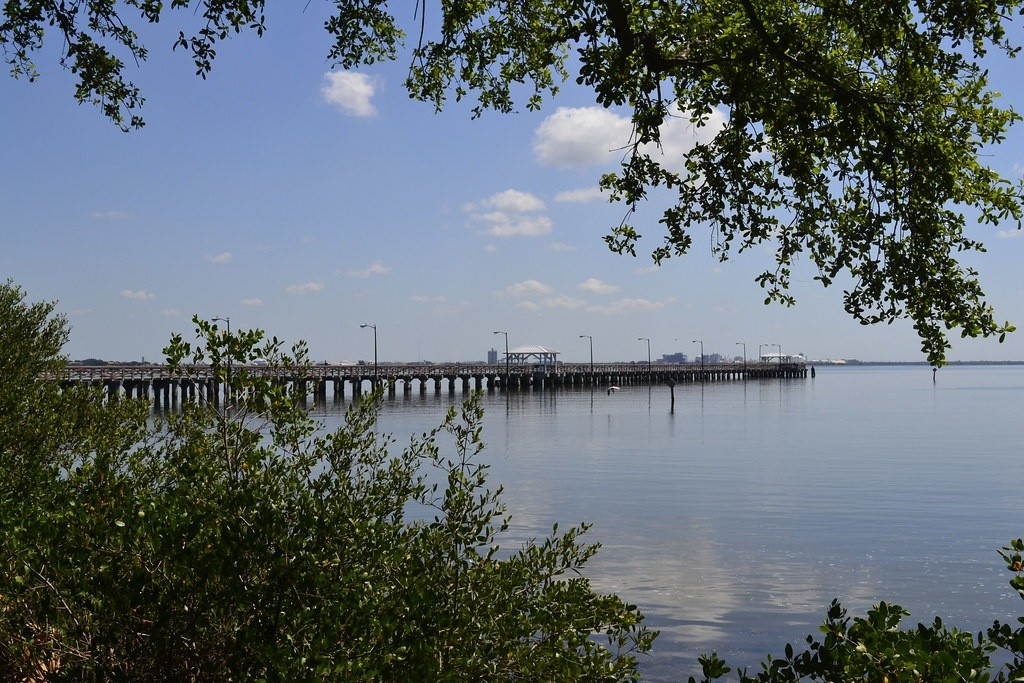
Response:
[[607, 386, 620, 396]]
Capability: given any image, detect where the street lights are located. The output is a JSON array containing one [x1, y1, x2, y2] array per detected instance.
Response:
[[638, 338, 651, 371], [736, 342, 746, 368], [493, 331, 508, 374], [212, 317, 230, 370], [759, 344, 768, 365], [772, 344, 781, 367], [579, 336, 593, 370], [359, 324, 378, 379], [692, 340, 704, 371]]
